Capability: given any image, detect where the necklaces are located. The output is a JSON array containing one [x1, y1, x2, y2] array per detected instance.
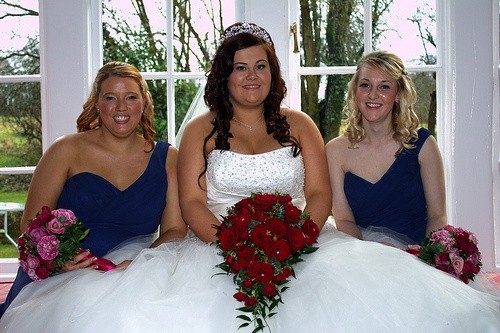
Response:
[[231, 111, 265, 134]]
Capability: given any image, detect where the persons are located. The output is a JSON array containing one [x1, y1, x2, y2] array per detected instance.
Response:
[[0, 61, 189, 319], [176, 23, 333, 244], [325, 51, 448, 249]]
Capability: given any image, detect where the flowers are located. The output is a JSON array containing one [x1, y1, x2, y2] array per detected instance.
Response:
[[399, 224, 483, 285], [210, 191, 320, 333], [18, 205, 117, 281]]
